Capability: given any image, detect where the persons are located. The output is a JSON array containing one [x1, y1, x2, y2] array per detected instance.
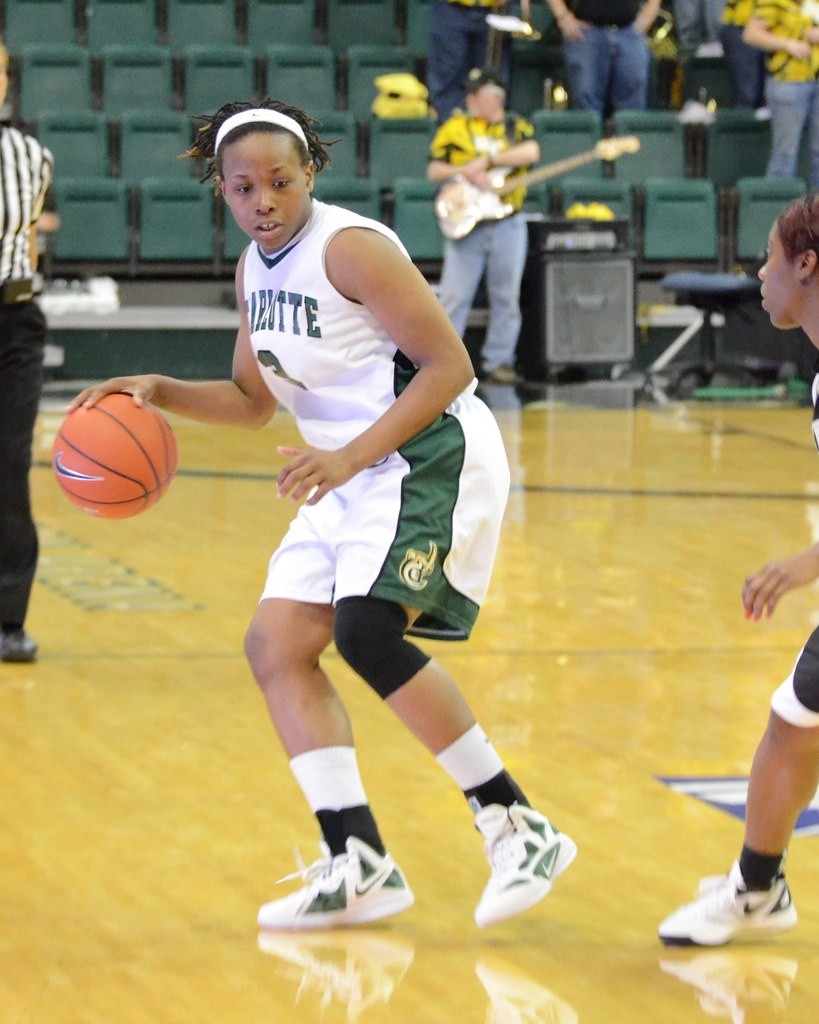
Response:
[[548, 0, 665, 119], [0, 37, 60, 663], [426, 80, 542, 384], [659, 190, 819, 949], [673, 0, 819, 180], [427, 0, 541, 124], [62, 97, 580, 930]]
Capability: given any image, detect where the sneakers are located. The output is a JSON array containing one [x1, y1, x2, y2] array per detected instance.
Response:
[[258, 928, 415, 1006], [473, 955, 578, 1024], [658, 947, 799, 1011], [657, 860, 796, 946], [473, 802, 577, 926], [256, 837, 411, 928]]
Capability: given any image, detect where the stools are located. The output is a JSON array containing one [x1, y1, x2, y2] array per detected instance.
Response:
[[661, 270, 761, 395]]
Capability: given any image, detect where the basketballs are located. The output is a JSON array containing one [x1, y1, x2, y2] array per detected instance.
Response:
[[52, 393, 177, 520]]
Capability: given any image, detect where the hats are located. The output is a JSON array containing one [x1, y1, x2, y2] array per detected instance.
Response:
[[460, 66, 504, 94]]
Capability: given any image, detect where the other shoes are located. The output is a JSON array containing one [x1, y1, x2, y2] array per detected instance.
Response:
[[490, 365, 518, 382], [0, 630, 40, 661]]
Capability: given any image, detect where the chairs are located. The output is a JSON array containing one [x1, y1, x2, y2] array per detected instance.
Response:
[[1, 0, 819, 279]]
